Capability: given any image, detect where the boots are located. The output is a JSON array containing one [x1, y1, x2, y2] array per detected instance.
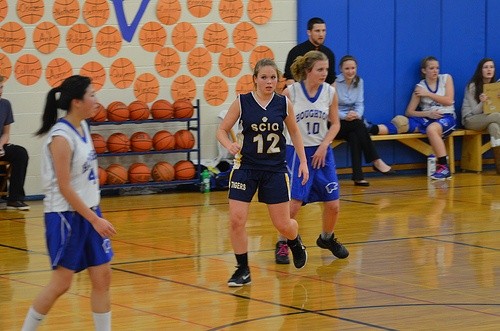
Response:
[[492, 144, 500, 175]]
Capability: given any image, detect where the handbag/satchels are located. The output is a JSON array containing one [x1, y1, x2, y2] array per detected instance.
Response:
[[193, 159, 232, 190]]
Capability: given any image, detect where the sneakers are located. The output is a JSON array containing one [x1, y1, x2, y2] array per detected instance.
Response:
[[275, 239, 290, 264], [229, 284, 252, 297], [276, 263, 290, 280], [316, 232, 349, 260], [227, 264, 252, 286], [317, 260, 350, 276], [430, 179, 450, 191], [286, 235, 308, 270], [430, 163, 451, 180], [289, 284, 307, 312]]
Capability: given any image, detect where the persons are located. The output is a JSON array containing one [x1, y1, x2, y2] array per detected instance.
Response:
[[22, 75, 116, 331], [275, 50, 349, 264], [215, 58, 310, 287], [328, 54, 394, 186], [462, 58, 500, 174], [364, 56, 457, 181], [0, 75, 31, 211], [282, 18, 337, 87]]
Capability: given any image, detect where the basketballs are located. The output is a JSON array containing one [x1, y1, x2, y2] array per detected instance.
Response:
[[175, 161, 195, 179], [175, 130, 195, 149], [173, 99, 193, 118], [106, 164, 129, 183], [128, 163, 151, 182], [153, 130, 175, 150], [107, 133, 130, 153], [150, 99, 174, 119], [152, 161, 175, 181], [107, 101, 130, 121], [128, 100, 150, 120], [90, 133, 106, 153], [89, 103, 108, 122], [98, 166, 107, 185], [131, 132, 152, 152]]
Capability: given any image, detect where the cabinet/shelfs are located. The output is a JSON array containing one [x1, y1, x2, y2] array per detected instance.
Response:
[[84, 97, 208, 198]]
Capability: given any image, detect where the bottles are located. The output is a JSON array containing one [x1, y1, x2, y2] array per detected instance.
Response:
[[427, 154, 436, 177], [201, 170, 210, 193]]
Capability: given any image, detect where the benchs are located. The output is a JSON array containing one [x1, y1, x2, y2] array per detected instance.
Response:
[[460, 128, 493, 174], [330, 128, 466, 178]]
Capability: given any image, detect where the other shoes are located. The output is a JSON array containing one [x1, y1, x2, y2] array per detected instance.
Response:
[[373, 165, 393, 176], [355, 180, 369, 186], [6, 200, 31, 211]]
